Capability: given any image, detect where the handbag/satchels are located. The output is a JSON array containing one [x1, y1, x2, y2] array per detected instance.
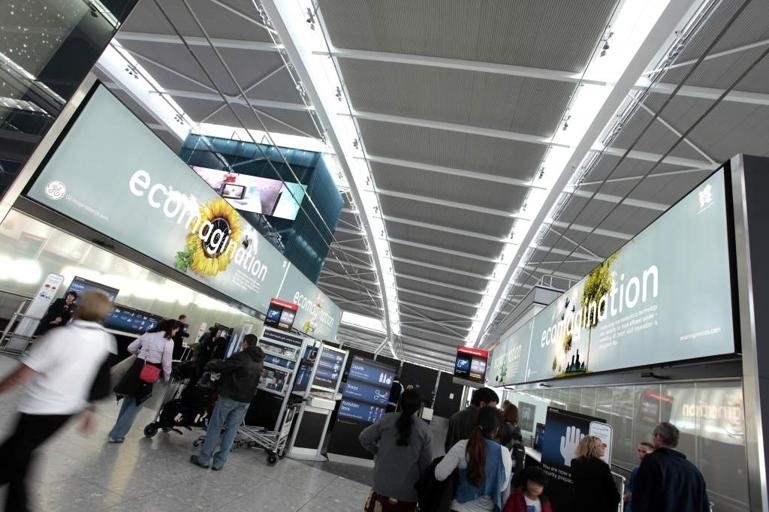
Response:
[[139, 364, 162, 383]]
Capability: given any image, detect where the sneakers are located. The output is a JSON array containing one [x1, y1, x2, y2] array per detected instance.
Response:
[[109, 435, 125, 443], [190, 454, 223, 472]]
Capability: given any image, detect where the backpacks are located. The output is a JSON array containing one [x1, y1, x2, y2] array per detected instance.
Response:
[[502, 422, 527, 473]]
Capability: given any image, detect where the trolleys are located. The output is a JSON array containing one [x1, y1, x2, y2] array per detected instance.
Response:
[[141, 333, 196, 440], [188, 325, 328, 468]]
